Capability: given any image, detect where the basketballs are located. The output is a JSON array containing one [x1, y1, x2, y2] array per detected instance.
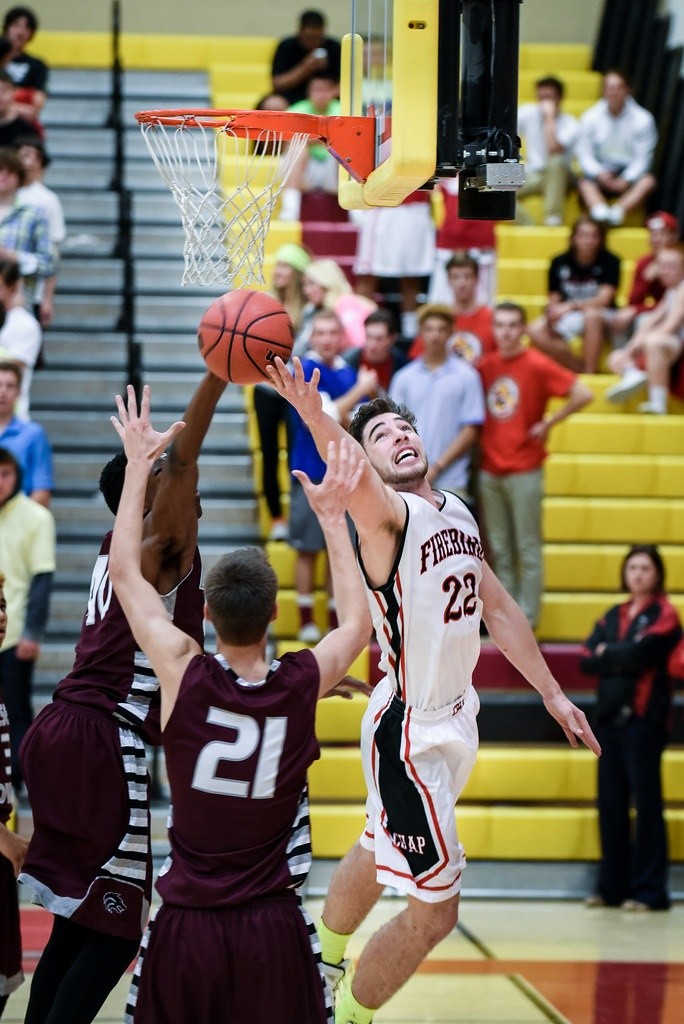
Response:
[[198, 289, 295, 386]]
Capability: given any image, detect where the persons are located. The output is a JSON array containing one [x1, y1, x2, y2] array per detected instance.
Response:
[[260, 237, 597, 641], [248, 10, 500, 311], [510, 66, 684, 415], [15, 373, 225, 1024], [577, 542, 683, 914], [108, 383, 373, 1024], [0, 573, 26, 1015], [262, 357, 600, 1023], [1, 444, 56, 819], [1, 5, 67, 506]]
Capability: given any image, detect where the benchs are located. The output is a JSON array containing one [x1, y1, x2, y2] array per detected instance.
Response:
[[23, 29, 684, 862]]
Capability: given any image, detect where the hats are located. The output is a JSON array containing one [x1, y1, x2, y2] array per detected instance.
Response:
[[648, 211, 679, 235]]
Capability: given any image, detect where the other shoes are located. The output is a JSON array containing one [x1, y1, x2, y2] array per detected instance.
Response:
[[321, 958, 347, 1010], [607, 369, 647, 404], [270, 521, 291, 540], [298, 623, 322, 643], [622, 900, 649, 912], [588, 894, 604, 906], [640, 394, 668, 415]]
